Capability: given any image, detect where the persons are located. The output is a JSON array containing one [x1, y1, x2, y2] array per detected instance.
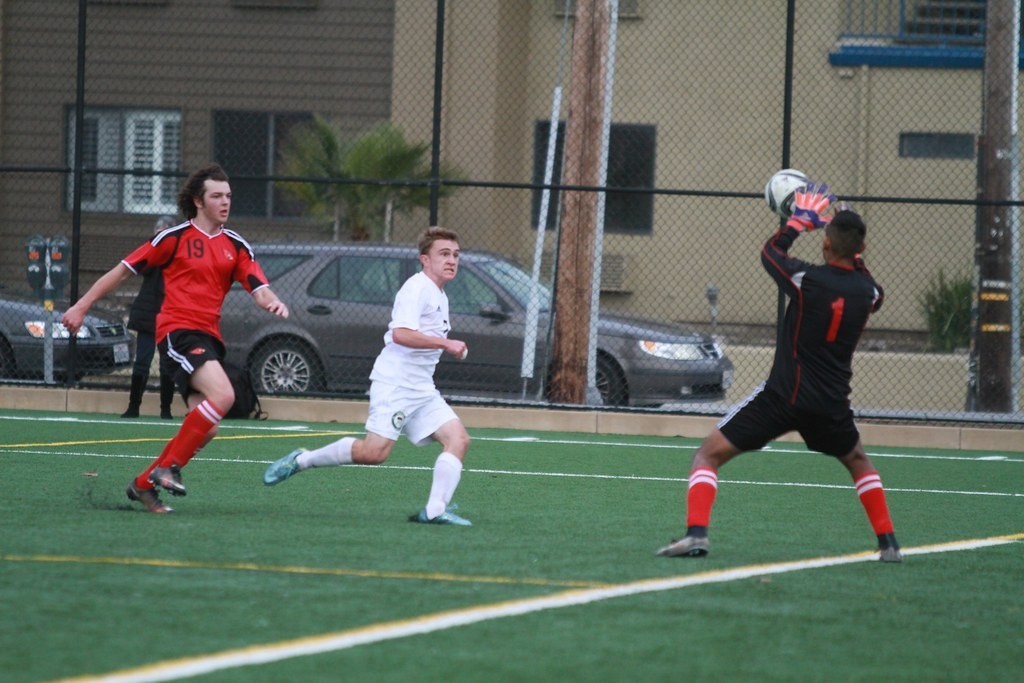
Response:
[[656, 180, 902, 563], [120, 215, 178, 419], [59, 166, 289, 514], [262, 227, 471, 527]]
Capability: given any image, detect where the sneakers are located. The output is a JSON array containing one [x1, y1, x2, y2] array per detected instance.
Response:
[[148, 464, 187, 497], [417, 507, 472, 528], [880, 547, 903, 564], [263, 447, 308, 485], [126, 478, 173, 514], [657, 536, 710, 558]]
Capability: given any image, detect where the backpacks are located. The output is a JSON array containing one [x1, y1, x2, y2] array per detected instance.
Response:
[[224, 363, 268, 420]]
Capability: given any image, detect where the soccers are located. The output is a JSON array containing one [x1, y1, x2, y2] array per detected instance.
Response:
[[764, 169, 810, 220]]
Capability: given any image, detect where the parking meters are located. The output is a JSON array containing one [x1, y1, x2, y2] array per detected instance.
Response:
[[26, 235, 68, 386], [705, 285, 720, 336]]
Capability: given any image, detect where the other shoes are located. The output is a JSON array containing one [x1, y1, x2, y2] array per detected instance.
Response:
[[161, 406, 172, 419], [121, 405, 138, 417]]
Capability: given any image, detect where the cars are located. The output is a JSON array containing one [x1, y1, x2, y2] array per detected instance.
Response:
[[1, 293, 137, 386], [221, 244, 734, 407]]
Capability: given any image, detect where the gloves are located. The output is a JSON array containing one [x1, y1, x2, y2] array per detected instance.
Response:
[[786, 179, 833, 233], [834, 201, 855, 214]]
